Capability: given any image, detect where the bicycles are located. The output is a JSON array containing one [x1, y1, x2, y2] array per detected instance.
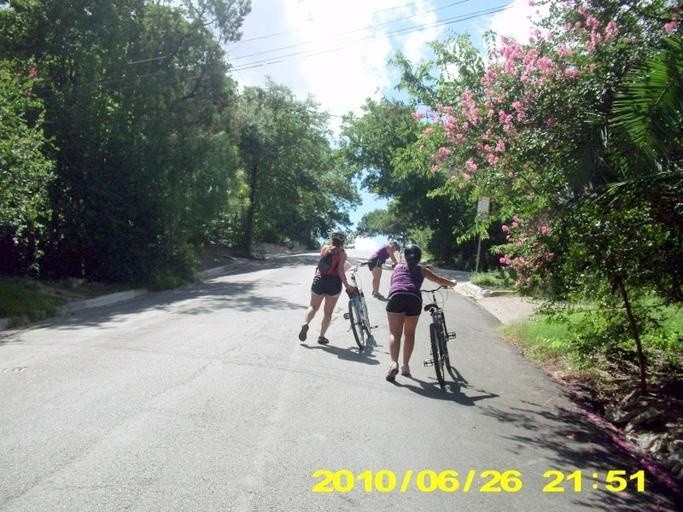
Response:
[[420, 286, 458, 388], [344, 262, 378, 350]]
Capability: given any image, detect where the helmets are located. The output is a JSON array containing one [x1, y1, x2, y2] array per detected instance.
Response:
[[332, 230, 347, 243], [391, 240, 400, 251], [404, 245, 421, 262]]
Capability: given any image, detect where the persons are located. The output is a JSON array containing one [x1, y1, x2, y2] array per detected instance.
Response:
[[367, 240, 401, 298], [384, 243, 458, 380], [298, 230, 357, 344]]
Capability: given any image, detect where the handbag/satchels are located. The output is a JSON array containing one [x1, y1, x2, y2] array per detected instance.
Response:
[[319, 254, 332, 273]]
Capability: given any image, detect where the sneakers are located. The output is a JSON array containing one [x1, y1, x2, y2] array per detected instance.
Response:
[[318, 335, 330, 344], [371, 290, 383, 299], [299, 325, 309, 341], [386, 362, 398, 380], [402, 366, 410, 376]]
